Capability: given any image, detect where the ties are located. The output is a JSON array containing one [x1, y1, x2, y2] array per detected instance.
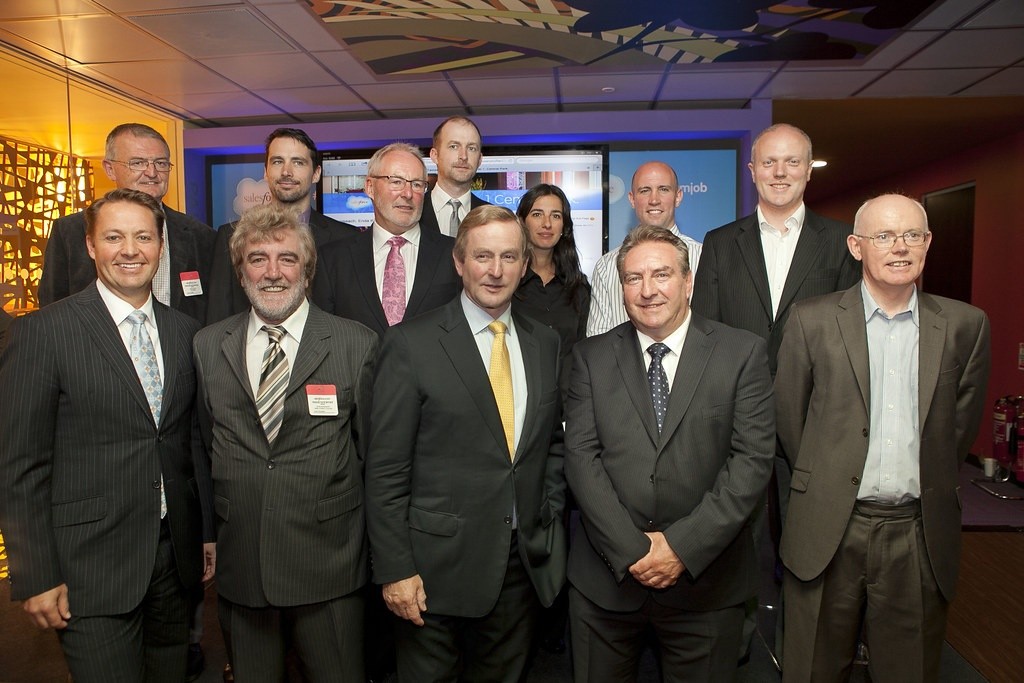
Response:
[[256, 326, 289, 450], [447, 199, 462, 238], [382, 237, 407, 327], [126, 310, 167, 520], [646, 343, 671, 439], [487, 320, 515, 464]]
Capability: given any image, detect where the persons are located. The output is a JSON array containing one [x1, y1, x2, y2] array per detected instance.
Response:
[[585, 161, 703, 338], [775, 195, 991, 683], [689, 122, 863, 373], [37, 122, 216, 322], [418, 115, 514, 240], [193, 198, 385, 683], [310, 144, 464, 336], [1, 188, 218, 683], [562, 225, 778, 683], [366, 205, 568, 683], [510, 184, 591, 360], [207, 127, 359, 322]]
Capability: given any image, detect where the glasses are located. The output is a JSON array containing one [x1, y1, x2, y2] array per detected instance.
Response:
[[371, 174, 430, 194], [109, 159, 173, 172], [856, 230, 927, 249]]
